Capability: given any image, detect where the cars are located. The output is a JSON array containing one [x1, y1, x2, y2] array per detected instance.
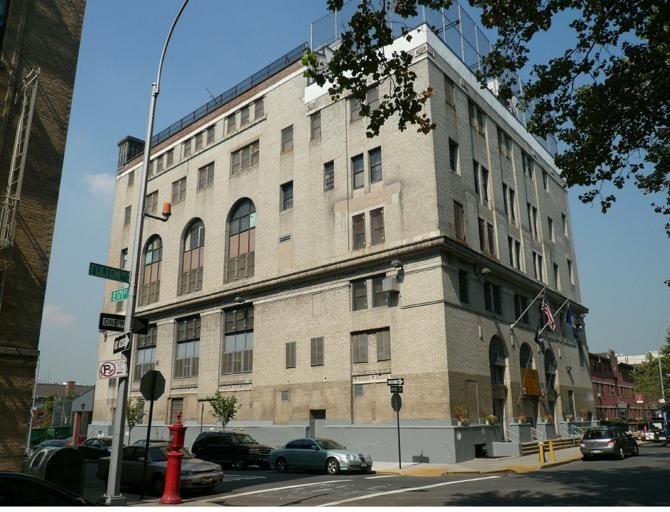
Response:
[[270, 437, 374, 474], [1, 436, 225, 504]]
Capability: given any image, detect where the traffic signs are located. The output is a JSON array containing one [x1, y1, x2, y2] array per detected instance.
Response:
[[113, 332, 132, 354], [99, 313, 149, 336], [387, 378, 404, 385]]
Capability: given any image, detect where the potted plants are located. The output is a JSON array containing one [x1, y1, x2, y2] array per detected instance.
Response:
[[454, 403, 588, 426]]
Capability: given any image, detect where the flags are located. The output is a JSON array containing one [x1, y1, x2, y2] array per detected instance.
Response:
[[564, 300, 576, 331], [539, 289, 556, 332]]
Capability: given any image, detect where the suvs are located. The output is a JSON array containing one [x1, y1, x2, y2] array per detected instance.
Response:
[[580, 425, 639, 459], [195, 431, 276, 467]]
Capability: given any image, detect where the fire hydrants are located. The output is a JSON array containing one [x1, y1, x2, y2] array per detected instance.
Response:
[[159, 410, 187, 503]]
